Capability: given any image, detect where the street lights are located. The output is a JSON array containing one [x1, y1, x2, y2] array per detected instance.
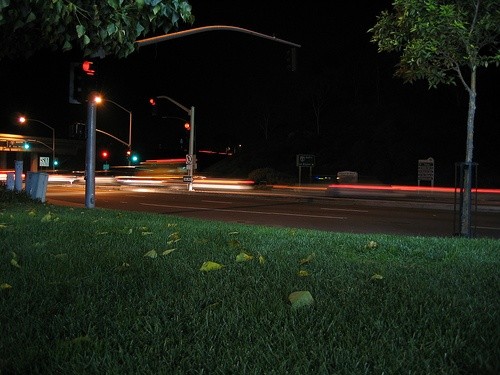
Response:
[[149, 96, 194, 191], [95, 97, 132, 166], [20, 118, 55, 174]]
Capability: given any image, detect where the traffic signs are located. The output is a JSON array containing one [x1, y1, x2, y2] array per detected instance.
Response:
[[69, 61, 89, 104], [103, 164, 109, 170], [183, 176, 191, 182]]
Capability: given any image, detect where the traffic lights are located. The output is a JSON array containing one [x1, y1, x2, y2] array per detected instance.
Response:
[[283, 47, 297, 74], [126, 151, 131, 160], [82, 57, 101, 101]]
